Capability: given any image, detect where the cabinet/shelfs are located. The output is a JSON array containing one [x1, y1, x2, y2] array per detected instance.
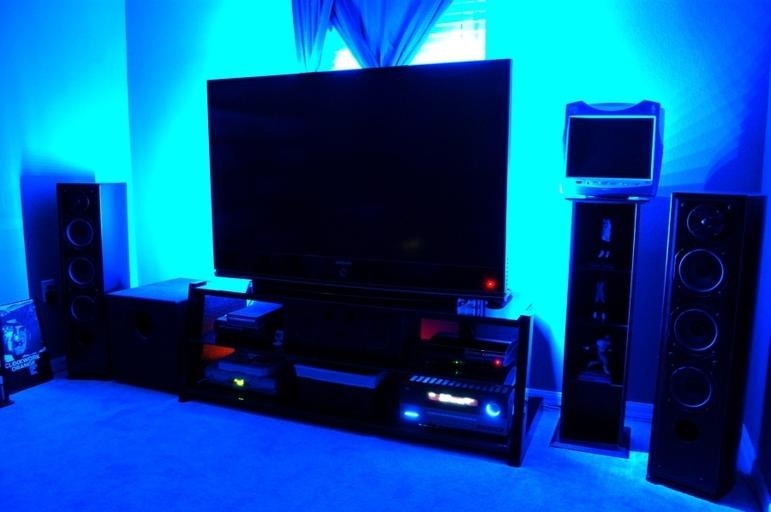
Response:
[[179, 280, 546, 468]]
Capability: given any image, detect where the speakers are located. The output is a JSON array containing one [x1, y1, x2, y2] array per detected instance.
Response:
[[54, 181, 131, 343], [645, 190, 765, 502]]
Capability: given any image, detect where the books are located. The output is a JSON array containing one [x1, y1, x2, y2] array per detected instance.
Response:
[[226, 302, 287, 324], [215, 348, 288, 377]]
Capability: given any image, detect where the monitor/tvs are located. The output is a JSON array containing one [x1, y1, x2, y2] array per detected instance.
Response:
[[205, 57, 514, 303], [559, 99, 667, 205]]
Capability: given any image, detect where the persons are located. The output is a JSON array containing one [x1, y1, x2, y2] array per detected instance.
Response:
[[1, 313, 34, 359]]
